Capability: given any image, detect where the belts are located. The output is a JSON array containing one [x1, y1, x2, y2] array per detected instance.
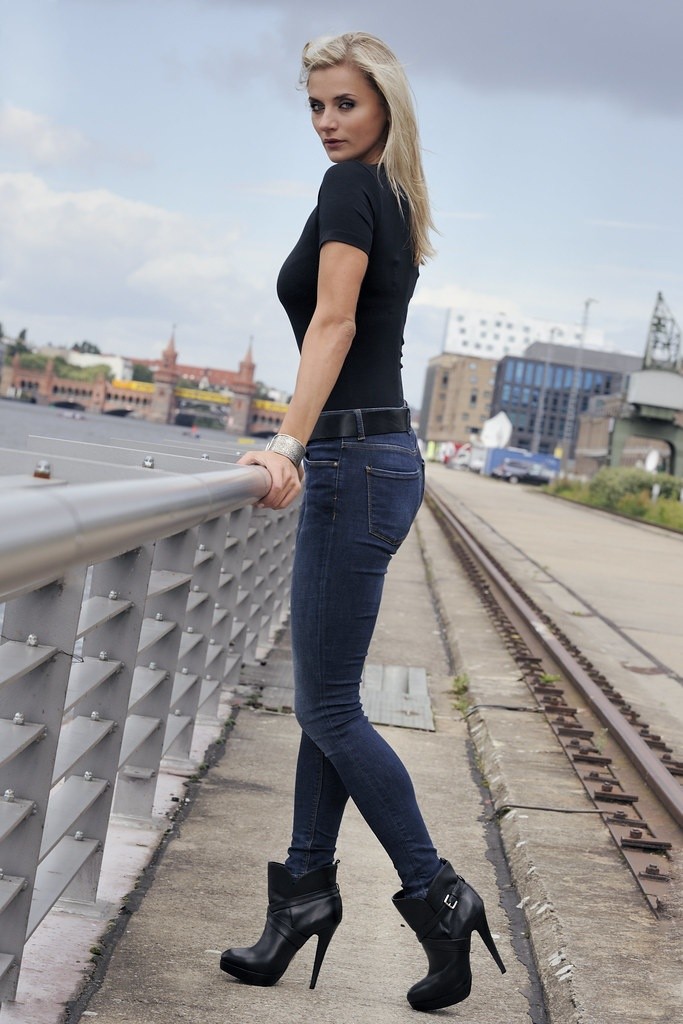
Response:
[[308, 409, 408, 441]]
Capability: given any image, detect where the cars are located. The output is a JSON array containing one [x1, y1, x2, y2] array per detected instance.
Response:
[[491, 455, 552, 485]]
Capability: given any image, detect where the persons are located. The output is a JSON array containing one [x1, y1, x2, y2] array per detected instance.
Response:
[[220, 30, 507, 1013]]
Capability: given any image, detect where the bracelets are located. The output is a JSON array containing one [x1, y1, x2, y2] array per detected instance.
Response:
[[265, 434, 306, 468]]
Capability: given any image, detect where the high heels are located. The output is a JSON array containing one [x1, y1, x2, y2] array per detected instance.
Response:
[[220, 859, 343, 990], [391, 858, 507, 1011]]
